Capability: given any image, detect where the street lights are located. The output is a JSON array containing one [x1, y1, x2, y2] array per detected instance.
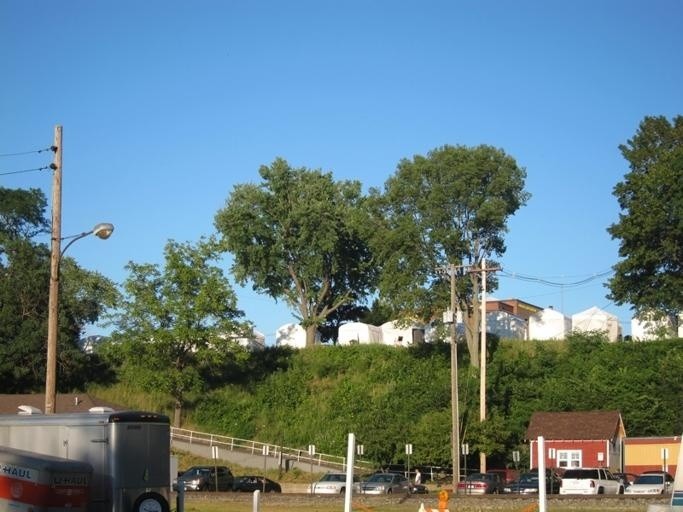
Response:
[[44, 221, 113, 414]]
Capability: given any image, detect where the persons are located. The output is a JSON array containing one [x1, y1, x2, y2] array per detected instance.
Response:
[[414, 468, 421, 484]]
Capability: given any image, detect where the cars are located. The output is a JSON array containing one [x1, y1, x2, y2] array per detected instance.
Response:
[[456, 467, 673, 497], [410, 484, 428, 494], [356, 472, 412, 494], [173, 465, 283, 493], [307, 471, 362, 495]]
